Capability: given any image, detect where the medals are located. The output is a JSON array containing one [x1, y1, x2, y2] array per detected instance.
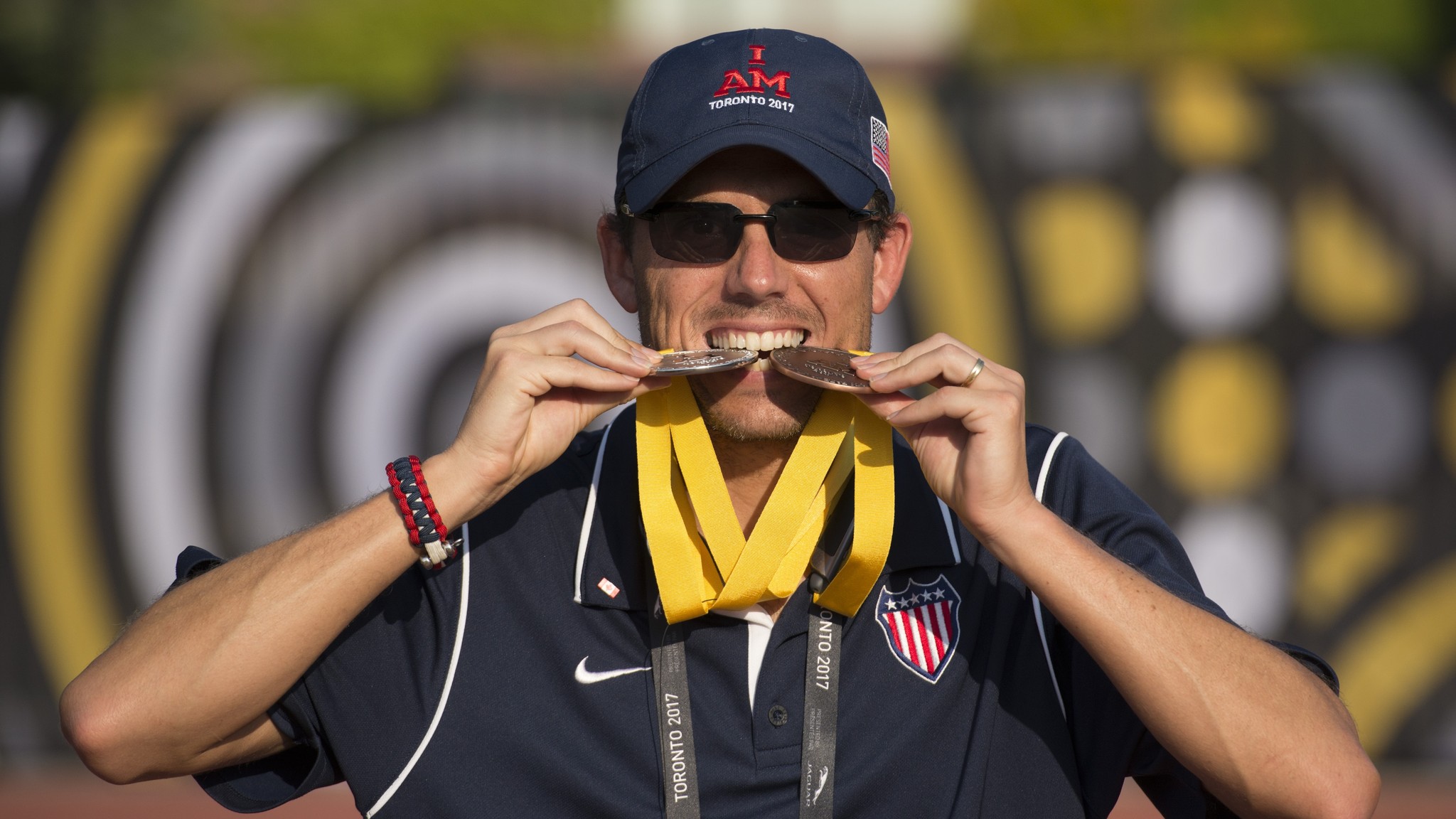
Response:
[[771, 346, 880, 396], [646, 349, 758, 377]]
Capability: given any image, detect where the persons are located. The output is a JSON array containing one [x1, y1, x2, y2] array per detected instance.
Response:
[[57, 27, 1384, 819]]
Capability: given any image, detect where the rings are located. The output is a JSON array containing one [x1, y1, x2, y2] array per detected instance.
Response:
[[957, 358, 986, 388]]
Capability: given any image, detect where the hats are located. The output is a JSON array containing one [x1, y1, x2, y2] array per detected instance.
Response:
[[616, 28, 894, 213]]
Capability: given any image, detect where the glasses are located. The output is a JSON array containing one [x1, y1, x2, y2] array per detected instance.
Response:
[[633, 195, 885, 263]]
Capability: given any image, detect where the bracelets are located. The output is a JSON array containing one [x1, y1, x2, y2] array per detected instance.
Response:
[[384, 454, 465, 571]]
